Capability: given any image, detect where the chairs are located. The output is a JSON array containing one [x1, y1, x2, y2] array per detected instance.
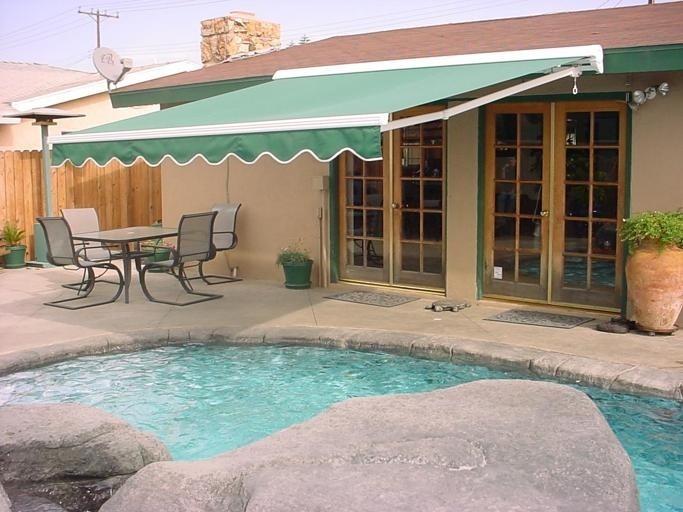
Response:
[[35, 202, 245, 310]]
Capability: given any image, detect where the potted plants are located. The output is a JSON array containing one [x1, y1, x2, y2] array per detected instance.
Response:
[[615, 208, 682, 331], [0, 218, 28, 269]]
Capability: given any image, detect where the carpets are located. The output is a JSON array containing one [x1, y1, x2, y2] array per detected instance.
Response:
[[322, 288, 422, 308], [483, 309, 597, 329]]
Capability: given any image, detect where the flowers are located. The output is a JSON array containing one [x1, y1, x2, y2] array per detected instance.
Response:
[[275, 235, 312, 270]]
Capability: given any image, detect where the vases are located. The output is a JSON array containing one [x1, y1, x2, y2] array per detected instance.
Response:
[[281, 257, 314, 290]]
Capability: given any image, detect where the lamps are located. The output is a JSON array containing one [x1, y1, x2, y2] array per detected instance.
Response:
[[627, 81, 670, 112]]
